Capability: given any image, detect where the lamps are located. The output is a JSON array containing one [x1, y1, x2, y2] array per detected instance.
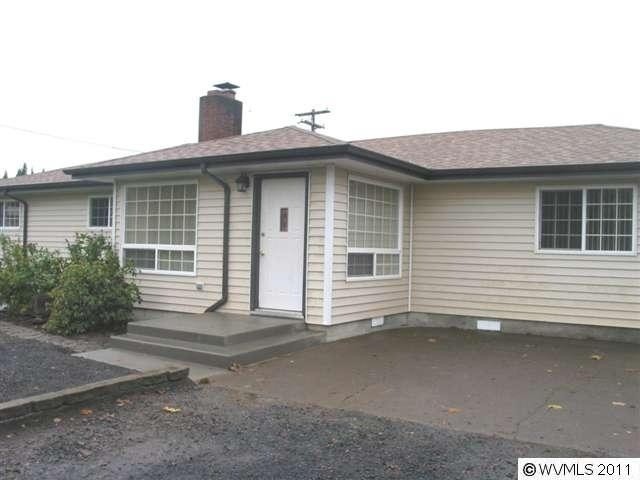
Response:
[[235, 171, 249, 192]]
